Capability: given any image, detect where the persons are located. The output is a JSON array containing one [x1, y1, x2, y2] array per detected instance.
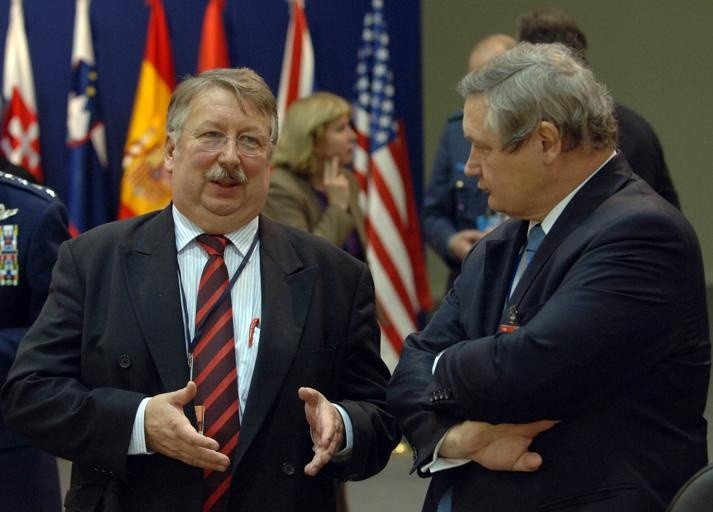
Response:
[[0, 171, 71, 512], [423, 33, 509, 286], [0, 68, 403, 512], [518, 13, 681, 208], [387, 41, 710, 511], [261, 91, 368, 263]]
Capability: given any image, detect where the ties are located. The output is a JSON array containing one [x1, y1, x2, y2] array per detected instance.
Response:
[[509, 225, 546, 299], [192, 234, 241, 511]]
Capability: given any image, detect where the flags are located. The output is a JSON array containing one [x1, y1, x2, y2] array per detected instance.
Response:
[[117, 2, 176, 222], [196, 1, 230, 72], [277, 0, 316, 136], [350, 0, 435, 454], [1, 1, 45, 188], [64, 0, 113, 239]]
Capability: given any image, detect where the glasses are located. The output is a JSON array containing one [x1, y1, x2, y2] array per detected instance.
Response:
[[176, 124, 273, 159]]
